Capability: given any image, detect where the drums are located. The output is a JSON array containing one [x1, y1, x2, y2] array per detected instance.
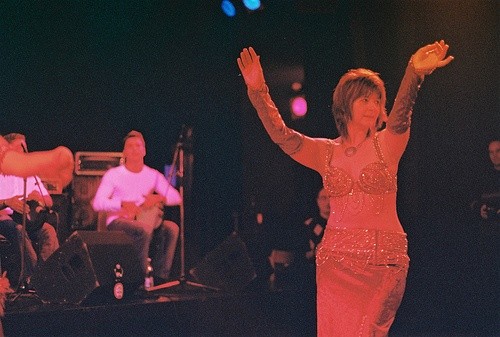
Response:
[[12, 200, 47, 233], [135, 203, 162, 229]]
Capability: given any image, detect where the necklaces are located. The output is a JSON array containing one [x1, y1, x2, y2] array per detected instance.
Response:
[[339, 136, 369, 156]]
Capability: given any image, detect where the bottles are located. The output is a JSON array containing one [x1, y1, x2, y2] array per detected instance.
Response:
[[111, 264, 125, 304], [144, 257, 154, 288]]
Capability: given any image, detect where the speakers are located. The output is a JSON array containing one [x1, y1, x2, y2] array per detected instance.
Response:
[[31, 230, 145, 306], [189, 231, 264, 296]]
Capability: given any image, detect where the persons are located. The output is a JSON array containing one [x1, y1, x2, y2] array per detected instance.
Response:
[[91, 131, 182, 291], [293, 188, 331, 285], [469, 139, 500, 298], [237, 39, 454, 336], [0, 131, 75, 294]]
